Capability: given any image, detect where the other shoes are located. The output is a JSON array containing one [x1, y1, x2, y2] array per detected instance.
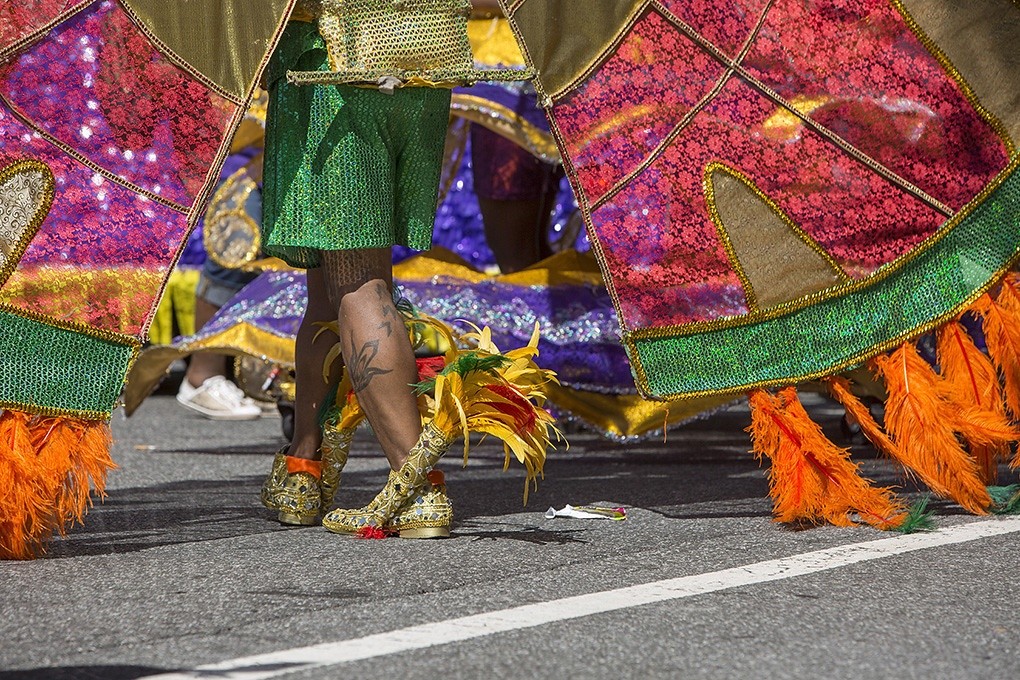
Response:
[[175, 375, 262, 420], [261, 444, 323, 524], [323, 459, 453, 538]]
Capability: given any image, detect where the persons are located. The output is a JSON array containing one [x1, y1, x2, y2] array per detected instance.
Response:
[[175, 256, 262, 422], [259, 0, 474, 537], [470, 121, 584, 435]]
